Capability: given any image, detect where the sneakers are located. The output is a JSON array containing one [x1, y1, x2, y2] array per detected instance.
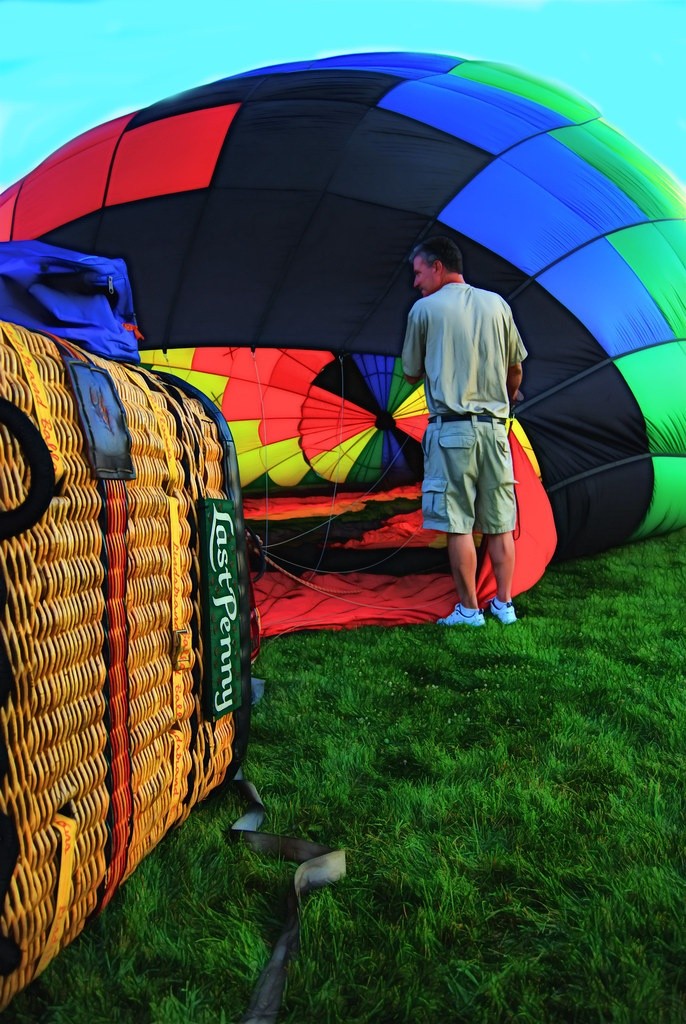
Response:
[[488, 597, 517, 625], [436, 603, 486, 628]]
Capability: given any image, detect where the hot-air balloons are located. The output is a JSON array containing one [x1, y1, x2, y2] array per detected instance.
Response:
[[0, 51, 684, 1024]]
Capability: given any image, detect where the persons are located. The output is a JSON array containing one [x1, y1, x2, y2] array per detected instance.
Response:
[[401, 235, 528, 627]]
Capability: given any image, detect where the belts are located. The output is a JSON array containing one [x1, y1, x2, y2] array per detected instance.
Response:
[[427, 415, 507, 425]]
